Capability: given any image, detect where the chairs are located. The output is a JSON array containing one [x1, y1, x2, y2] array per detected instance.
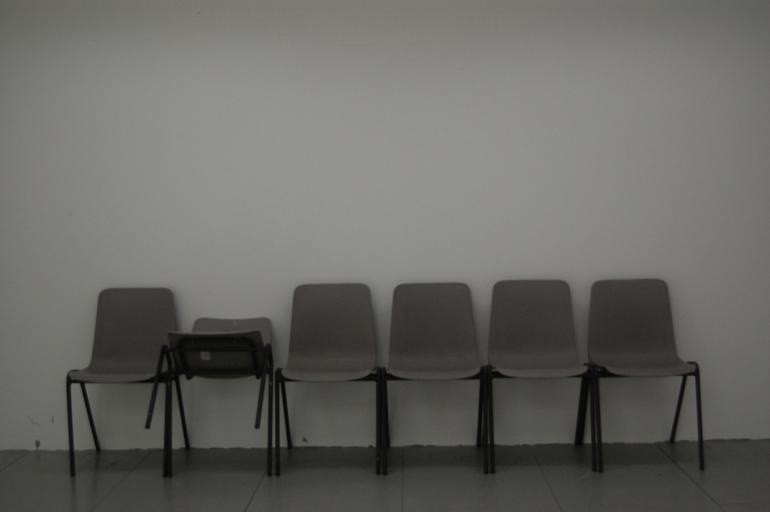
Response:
[[146, 318, 272, 478], [65, 288, 189, 478], [382, 283, 488, 476], [581, 279, 704, 472], [275, 283, 382, 477], [488, 280, 597, 473]]
